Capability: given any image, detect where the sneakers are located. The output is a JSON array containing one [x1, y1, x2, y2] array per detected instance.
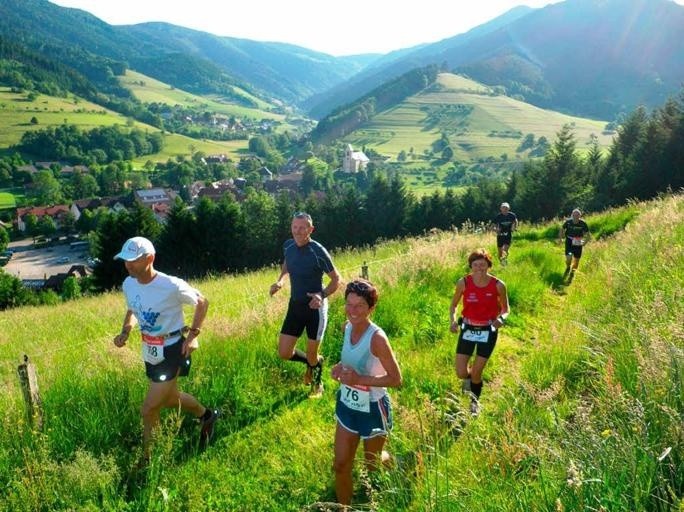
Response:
[[462, 378, 472, 396], [201, 409, 219, 440], [564, 266, 574, 283], [307, 381, 324, 400], [469, 400, 478, 417], [304, 366, 313, 386]]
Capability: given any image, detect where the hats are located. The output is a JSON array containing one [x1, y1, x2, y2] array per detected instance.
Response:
[[114, 236, 156, 262], [501, 202, 510, 208]]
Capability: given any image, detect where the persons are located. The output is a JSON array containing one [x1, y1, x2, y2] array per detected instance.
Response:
[[112, 236, 221, 477], [447, 250, 510, 414], [560, 207, 592, 280], [488, 201, 518, 264], [269, 212, 342, 401], [330, 279, 404, 511]]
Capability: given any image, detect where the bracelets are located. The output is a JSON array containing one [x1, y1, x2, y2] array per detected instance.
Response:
[[189, 326, 201, 337], [121, 330, 129, 338], [498, 315, 505, 322]]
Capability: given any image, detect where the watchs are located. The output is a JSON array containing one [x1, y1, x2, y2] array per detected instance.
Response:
[[322, 290, 329, 298]]
